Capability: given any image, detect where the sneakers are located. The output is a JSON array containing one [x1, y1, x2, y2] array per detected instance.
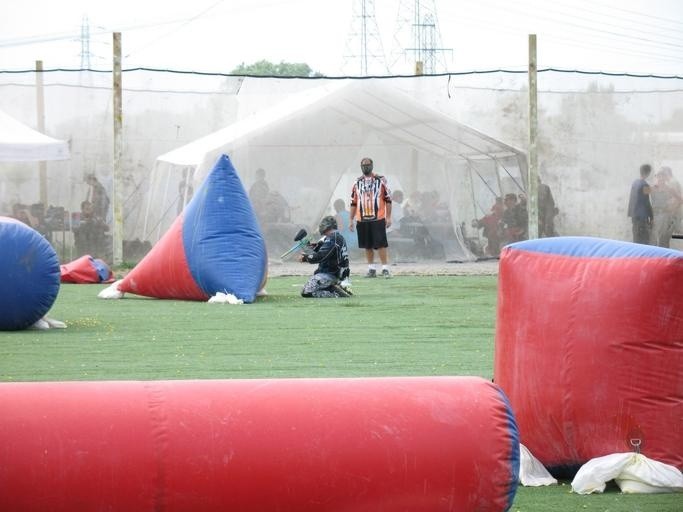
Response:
[[338, 283, 354, 295], [326, 285, 350, 298], [382, 270, 390, 279], [365, 269, 377, 278]]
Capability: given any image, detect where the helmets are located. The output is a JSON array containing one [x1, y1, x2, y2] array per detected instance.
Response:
[[318, 216, 338, 234]]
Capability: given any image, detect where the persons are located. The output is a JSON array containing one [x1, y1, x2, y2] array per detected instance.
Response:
[[249, 168, 269, 212], [625, 164, 653, 244], [332, 199, 359, 258], [14, 203, 47, 243], [655, 165, 680, 196], [348, 156, 392, 280], [386, 188, 454, 262], [473, 174, 556, 259], [177, 165, 193, 216], [298, 214, 350, 299], [81, 172, 110, 221], [75, 199, 112, 264], [648, 171, 681, 248]]
